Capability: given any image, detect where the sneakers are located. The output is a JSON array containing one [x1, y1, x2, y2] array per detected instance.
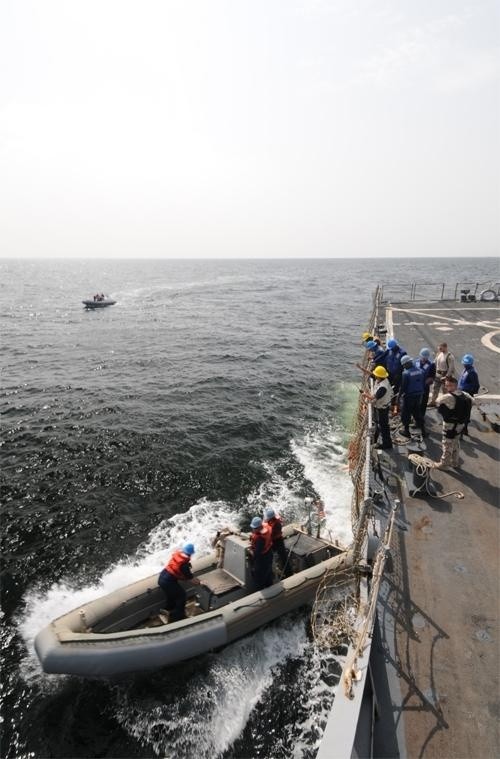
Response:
[[435, 463, 450, 472], [399, 429, 409, 437]]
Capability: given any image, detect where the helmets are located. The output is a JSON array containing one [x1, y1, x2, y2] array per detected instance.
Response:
[[250, 517, 263, 528], [362, 333, 473, 378], [183, 544, 195, 554], [266, 509, 275, 517]]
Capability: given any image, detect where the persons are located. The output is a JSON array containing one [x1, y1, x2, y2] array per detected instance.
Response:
[[93, 292, 108, 302], [158, 543, 203, 624], [266, 511, 294, 579], [248, 518, 276, 587], [356, 328, 480, 473]]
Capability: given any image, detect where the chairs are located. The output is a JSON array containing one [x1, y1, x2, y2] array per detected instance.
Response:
[[194, 533, 253, 612]]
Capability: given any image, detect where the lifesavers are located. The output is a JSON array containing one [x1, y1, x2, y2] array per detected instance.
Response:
[[480, 289, 496, 301]]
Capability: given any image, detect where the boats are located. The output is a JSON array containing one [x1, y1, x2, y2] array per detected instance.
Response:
[[81, 298, 116, 309], [34, 523, 357, 676]]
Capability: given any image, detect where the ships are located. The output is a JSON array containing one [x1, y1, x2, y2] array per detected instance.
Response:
[[317, 274, 499, 759]]
